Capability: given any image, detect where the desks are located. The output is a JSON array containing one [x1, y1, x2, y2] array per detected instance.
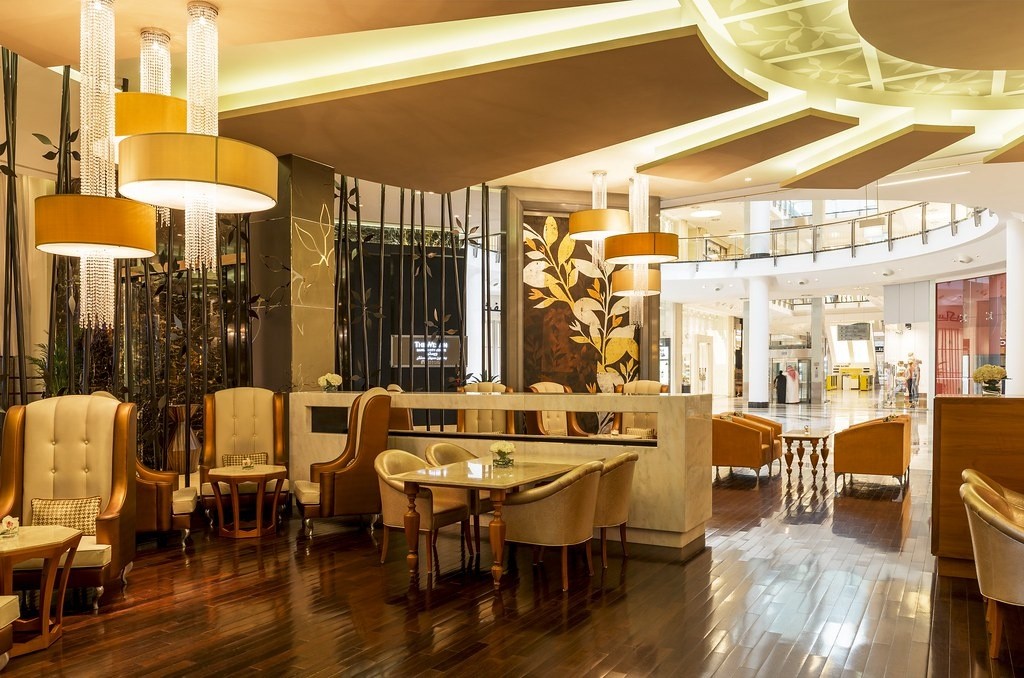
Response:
[[386, 453, 606, 593], [777, 429, 837, 488], [208, 464, 287, 539], [588, 433, 643, 439], [0, 524, 85, 659]]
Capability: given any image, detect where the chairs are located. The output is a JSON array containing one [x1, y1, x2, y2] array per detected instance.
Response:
[[387, 383, 414, 430], [425, 442, 495, 552], [456, 383, 515, 434], [958, 483, 1024, 659], [711, 413, 772, 490], [539, 450, 638, 569], [960, 474, 1024, 624], [525, 382, 595, 438], [91, 390, 197, 555], [498, 460, 605, 591], [0, 395, 138, 618], [374, 449, 475, 574], [722, 411, 783, 480], [834, 414, 911, 502], [293, 386, 392, 559], [612, 380, 670, 434], [198, 386, 290, 542]]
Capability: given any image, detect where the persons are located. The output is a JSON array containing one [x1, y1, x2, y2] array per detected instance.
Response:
[[905, 359, 920, 407]]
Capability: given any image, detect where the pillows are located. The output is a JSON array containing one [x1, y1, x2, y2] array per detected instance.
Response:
[[222, 452, 270, 467], [30, 495, 103, 536], [733, 411, 745, 418], [625, 426, 655, 439]]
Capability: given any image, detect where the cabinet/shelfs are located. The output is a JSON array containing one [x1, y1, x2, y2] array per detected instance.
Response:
[[933, 393, 1024, 580]]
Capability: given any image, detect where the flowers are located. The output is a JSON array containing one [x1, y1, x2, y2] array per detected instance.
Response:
[[317, 372, 343, 391], [973, 363, 1008, 391], [242, 458, 255, 467], [1, 514, 20, 529], [489, 440, 517, 458]]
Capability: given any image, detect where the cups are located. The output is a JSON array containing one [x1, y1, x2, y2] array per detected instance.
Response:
[[242, 460, 255, 472], [804, 426, 810, 434], [611, 429, 619, 439]]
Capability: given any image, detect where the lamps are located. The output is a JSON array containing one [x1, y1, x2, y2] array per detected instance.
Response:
[[87, 26, 187, 164], [605, 163, 681, 265], [612, 176, 662, 297], [35, 0, 157, 259], [117, 0, 280, 214], [569, 169, 633, 241]]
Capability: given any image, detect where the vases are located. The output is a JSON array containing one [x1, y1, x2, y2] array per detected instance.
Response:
[[242, 466, 256, 471], [0, 527, 19, 540], [493, 458, 515, 469], [981, 388, 1001, 397], [325, 384, 338, 393]]
